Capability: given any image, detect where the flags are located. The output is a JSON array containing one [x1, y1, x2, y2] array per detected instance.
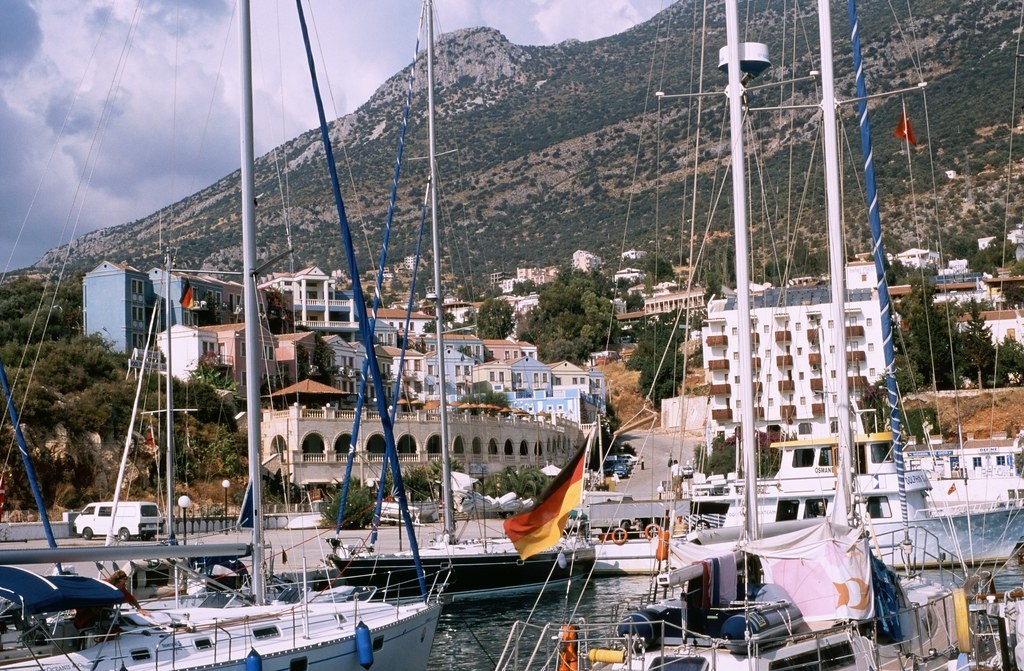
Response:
[[179, 277, 194, 310], [503, 422, 598, 561], [893, 103, 917, 147], [948, 482, 956, 496]]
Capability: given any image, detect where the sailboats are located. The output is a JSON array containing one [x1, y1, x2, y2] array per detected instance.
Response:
[[0, 1, 1024, 670]]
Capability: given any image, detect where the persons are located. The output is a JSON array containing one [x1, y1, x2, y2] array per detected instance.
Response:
[[671, 460, 684, 501], [73, 570, 151, 651], [641, 455, 645, 470]]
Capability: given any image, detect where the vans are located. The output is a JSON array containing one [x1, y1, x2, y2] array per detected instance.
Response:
[[71, 501, 162, 541]]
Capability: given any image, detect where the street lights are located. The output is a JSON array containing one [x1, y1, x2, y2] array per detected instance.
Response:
[[685, 462, 690, 490], [176, 495, 191, 545], [366, 481, 375, 500], [657, 486, 664, 499], [691, 458, 695, 471], [221, 478, 231, 535]]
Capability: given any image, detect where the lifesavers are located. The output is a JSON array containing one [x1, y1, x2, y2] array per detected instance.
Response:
[[610, 527, 629, 547], [644, 522, 664, 542]]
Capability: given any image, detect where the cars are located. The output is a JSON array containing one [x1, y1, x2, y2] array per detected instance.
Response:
[[681, 466, 693, 478]]
